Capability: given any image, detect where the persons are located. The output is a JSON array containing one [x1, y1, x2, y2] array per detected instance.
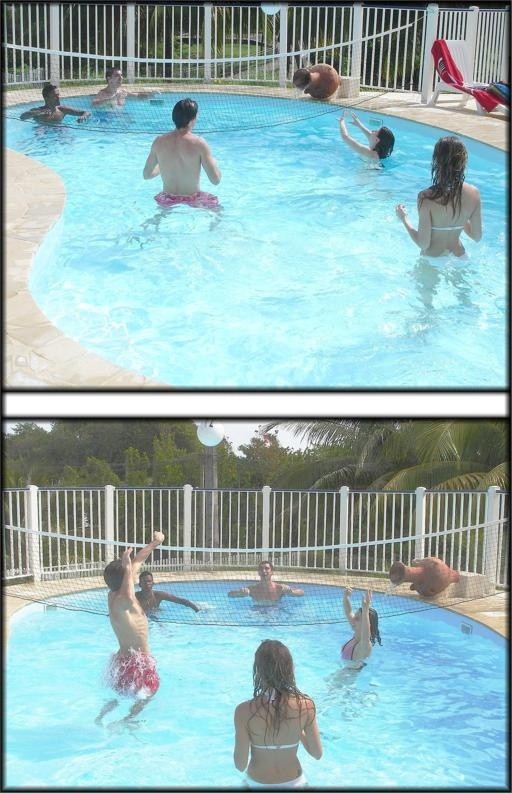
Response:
[[142, 97, 222, 229], [92, 530, 165, 733], [232, 637, 323, 790], [340, 584, 382, 670], [20, 84, 91, 130], [337, 108, 394, 171], [134, 571, 201, 621], [91, 68, 161, 113], [395, 136, 482, 309], [228, 561, 304, 609]]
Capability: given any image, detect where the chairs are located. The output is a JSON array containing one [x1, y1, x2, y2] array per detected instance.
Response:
[[423, 34, 507, 115]]
